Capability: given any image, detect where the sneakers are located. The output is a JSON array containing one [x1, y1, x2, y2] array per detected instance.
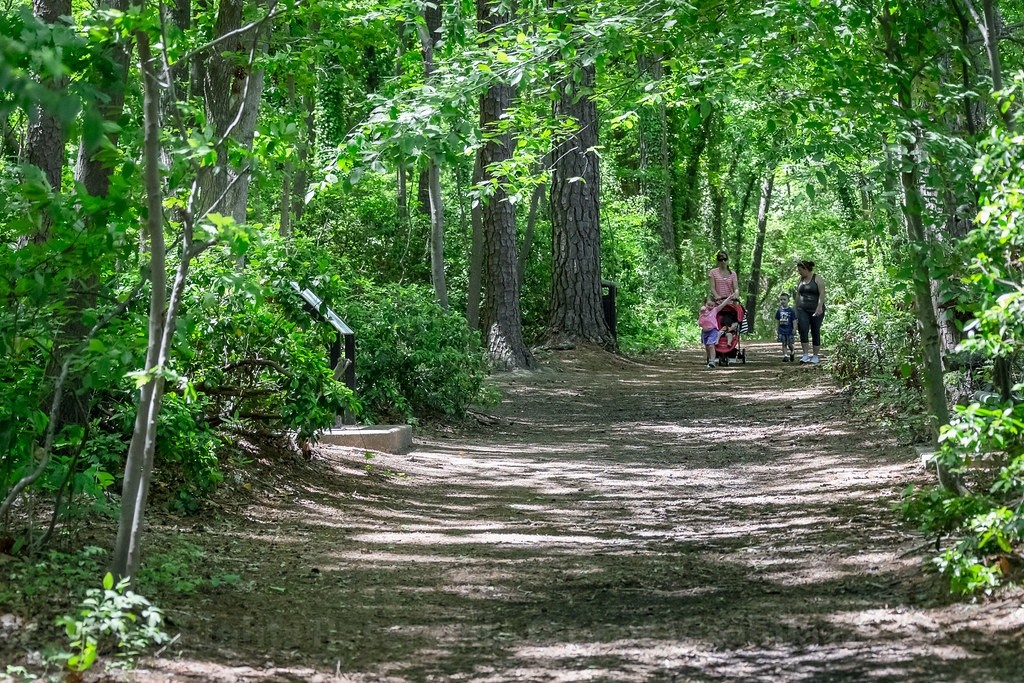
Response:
[[810, 354, 820, 364], [800, 354, 810, 363]]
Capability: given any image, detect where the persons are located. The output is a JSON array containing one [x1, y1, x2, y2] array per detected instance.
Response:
[[698, 292, 735, 367], [710, 251, 740, 368], [796, 260, 825, 364], [775, 293, 798, 362], [715, 322, 738, 347]]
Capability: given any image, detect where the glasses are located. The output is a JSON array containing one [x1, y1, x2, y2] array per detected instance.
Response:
[[718, 258, 727, 262]]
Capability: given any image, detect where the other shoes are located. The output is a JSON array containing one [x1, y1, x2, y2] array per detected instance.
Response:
[[709, 359, 716, 368], [707, 363, 710, 368], [790, 354, 795, 362], [782, 356, 788, 362]]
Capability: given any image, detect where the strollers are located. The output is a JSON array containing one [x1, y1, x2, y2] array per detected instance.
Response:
[[708, 297, 746, 365]]
